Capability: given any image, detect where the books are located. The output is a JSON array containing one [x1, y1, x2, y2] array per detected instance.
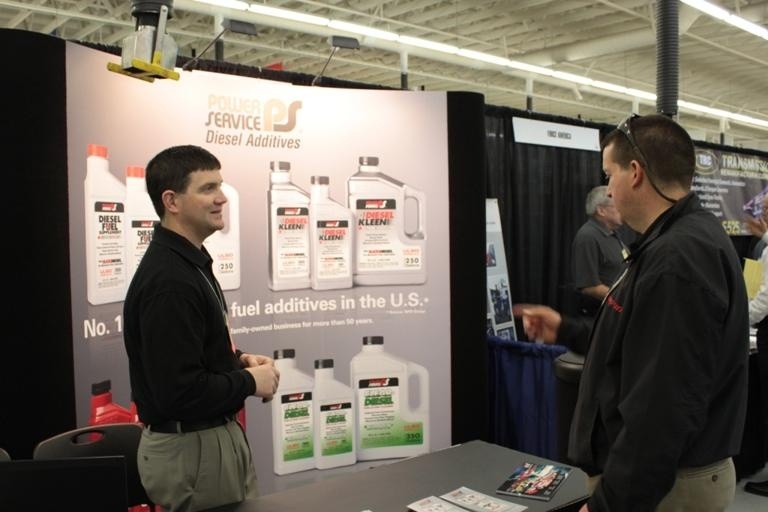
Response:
[[496, 463, 572, 502]]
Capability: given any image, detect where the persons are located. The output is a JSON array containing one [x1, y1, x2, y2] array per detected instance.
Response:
[[571, 184, 633, 298], [741, 192, 768, 497], [121, 146, 279, 510], [510, 112, 750, 512]]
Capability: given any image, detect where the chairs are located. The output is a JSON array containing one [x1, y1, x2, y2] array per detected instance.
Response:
[[32, 420, 156, 512]]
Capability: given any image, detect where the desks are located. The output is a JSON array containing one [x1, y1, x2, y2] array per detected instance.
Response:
[[200, 439, 593, 511]]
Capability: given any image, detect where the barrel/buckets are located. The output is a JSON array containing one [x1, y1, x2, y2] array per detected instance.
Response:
[[202, 181, 243, 293], [350, 335, 434, 464], [345, 155, 429, 289]]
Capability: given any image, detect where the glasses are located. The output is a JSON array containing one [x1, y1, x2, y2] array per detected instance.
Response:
[[617, 113, 649, 170]]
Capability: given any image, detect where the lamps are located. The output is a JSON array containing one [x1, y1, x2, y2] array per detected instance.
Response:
[[308, 32, 361, 88], [181, 14, 259, 72]]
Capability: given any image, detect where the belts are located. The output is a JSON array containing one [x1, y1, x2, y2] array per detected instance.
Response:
[[145, 411, 236, 434]]
